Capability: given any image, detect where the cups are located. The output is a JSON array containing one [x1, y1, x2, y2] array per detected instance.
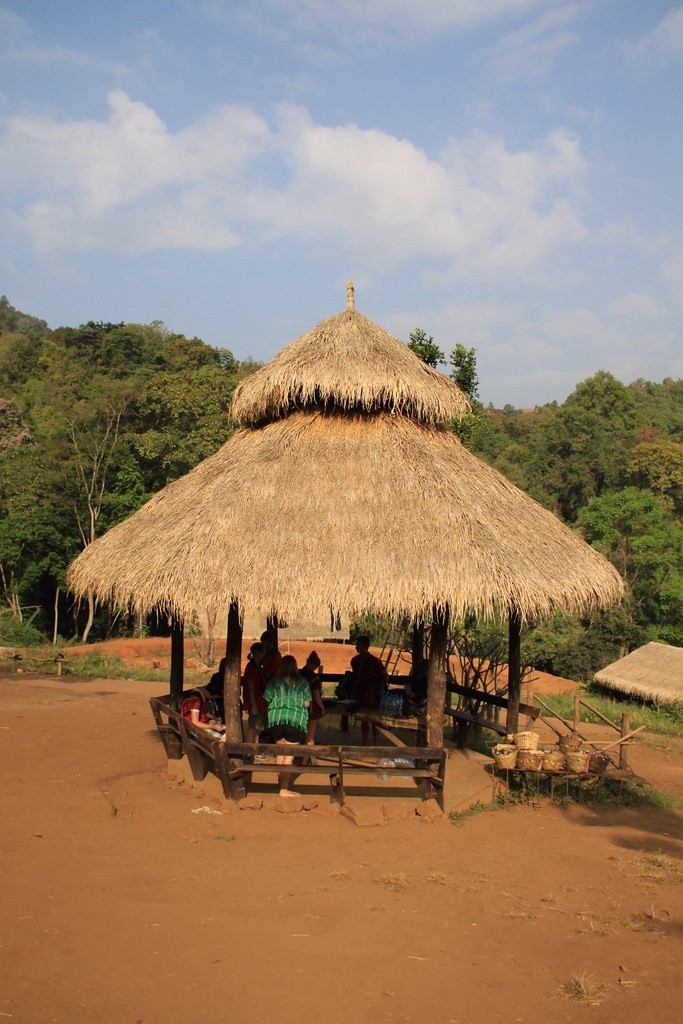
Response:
[[318, 666, 323, 673]]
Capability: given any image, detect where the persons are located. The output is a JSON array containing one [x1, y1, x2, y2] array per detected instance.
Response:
[[300, 651, 328, 766], [178, 672, 230, 743], [209, 657, 245, 745], [259, 630, 283, 677], [262, 655, 313, 799], [345, 635, 390, 747], [243, 641, 272, 765]]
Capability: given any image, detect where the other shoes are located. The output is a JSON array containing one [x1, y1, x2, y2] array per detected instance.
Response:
[[250, 753, 269, 762], [242, 754, 263, 765]]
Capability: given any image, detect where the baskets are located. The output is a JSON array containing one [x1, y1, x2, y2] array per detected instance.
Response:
[[566, 752, 591, 773], [513, 731, 540, 749], [517, 749, 544, 772], [492, 744, 518, 770], [560, 735, 583, 754], [589, 753, 610, 774], [542, 749, 566, 772]]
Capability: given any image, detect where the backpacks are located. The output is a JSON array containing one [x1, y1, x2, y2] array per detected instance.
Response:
[[169, 688, 193, 727], [335, 672, 352, 701]]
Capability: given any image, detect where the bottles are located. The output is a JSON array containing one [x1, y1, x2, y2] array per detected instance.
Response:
[[379, 758, 391, 780]]
